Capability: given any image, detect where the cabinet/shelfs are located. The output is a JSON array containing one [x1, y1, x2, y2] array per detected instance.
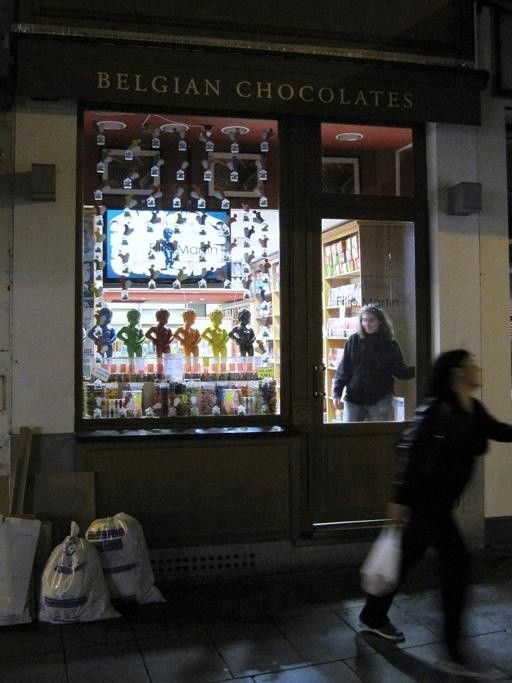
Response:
[[252, 221, 407, 420]]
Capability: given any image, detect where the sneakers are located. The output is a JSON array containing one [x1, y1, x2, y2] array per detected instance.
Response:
[[359, 618, 404, 641]]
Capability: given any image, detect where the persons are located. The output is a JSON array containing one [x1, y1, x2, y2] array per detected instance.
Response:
[[331, 305, 415, 419], [357, 348, 511, 678], [87, 307, 255, 381]]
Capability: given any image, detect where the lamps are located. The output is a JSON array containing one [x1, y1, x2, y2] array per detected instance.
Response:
[[448, 183, 482, 215], [31, 163, 56, 202]]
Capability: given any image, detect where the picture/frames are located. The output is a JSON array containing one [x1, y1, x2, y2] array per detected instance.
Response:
[[101, 149, 160, 196], [208, 152, 262, 199], [321, 157, 361, 195]]
